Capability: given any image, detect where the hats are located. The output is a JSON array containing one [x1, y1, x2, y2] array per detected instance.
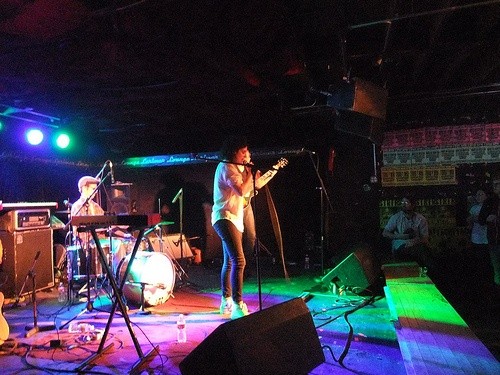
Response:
[[78, 177, 99, 189]]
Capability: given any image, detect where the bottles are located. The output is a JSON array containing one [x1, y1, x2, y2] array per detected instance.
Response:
[[177, 314, 186, 341], [58, 282, 65, 302], [69, 323, 94, 333], [305, 254, 310, 270]]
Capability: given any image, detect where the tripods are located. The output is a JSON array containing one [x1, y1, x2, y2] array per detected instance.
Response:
[[56, 168, 124, 329]]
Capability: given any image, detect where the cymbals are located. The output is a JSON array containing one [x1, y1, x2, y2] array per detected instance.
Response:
[[110, 182, 134, 186]]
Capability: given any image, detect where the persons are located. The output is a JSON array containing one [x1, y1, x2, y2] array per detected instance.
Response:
[[382, 194, 443, 266], [211, 135, 260, 319], [70, 176, 132, 245], [455, 176, 500, 286]]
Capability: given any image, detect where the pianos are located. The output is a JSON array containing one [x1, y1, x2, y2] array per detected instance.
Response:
[[71, 214, 159, 372]]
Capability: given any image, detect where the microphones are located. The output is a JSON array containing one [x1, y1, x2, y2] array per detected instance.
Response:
[[110, 161, 115, 183], [172, 187, 183, 202], [244, 159, 260, 173]]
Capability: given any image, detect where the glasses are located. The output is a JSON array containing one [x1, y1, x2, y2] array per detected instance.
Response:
[[401, 201, 410, 205]]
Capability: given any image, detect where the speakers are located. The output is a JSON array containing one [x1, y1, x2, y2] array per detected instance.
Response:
[[146, 231, 194, 264], [178, 296, 325, 375], [0, 227, 54, 300], [321, 252, 370, 295]]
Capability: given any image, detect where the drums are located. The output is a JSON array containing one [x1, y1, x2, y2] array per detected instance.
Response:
[[124, 237, 150, 255], [116, 250, 176, 306], [110, 186, 127, 202], [92, 237, 127, 274], [65, 245, 111, 281]]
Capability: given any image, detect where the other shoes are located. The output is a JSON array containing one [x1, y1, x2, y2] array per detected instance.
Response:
[[231, 303, 248, 319], [220, 299, 233, 319]]
